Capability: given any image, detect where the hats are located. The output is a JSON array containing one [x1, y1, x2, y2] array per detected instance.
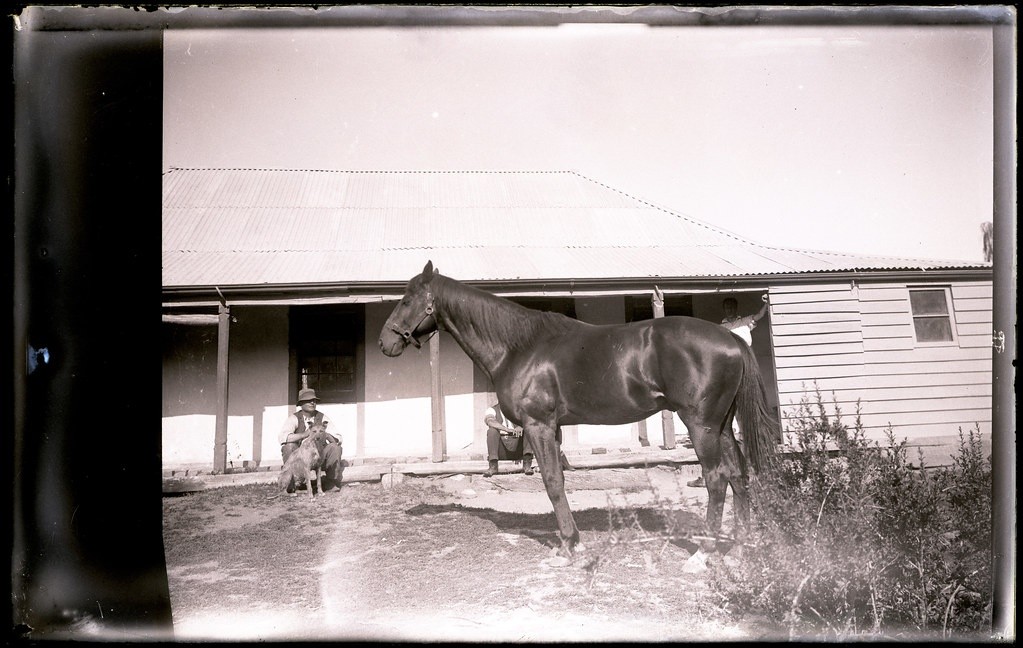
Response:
[[296, 388, 320, 407]]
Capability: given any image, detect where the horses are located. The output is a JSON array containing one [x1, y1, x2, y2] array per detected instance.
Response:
[[376, 259, 780, 577]]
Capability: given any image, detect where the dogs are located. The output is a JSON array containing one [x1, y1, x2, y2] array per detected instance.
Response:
[[266, 419, 328, 501]]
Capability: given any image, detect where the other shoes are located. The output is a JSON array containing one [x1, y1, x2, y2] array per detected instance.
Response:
[[327, 485, 340, 491], [687, 477, 706, 487]]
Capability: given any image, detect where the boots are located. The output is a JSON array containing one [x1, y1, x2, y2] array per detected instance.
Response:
[[523, 455, 533, 474], [484, 459, 498, 477]]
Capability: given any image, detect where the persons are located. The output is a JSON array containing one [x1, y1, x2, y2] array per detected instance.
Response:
[[278, 389, 343, 494], [483, 402, 534, 477], [721, 292, 768, 442]]
